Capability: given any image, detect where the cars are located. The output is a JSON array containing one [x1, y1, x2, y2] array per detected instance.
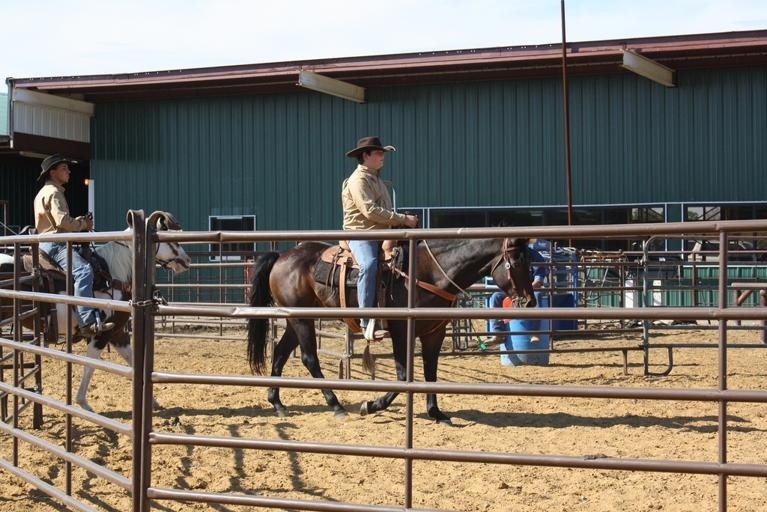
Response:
[[685, 241, 756, 264]]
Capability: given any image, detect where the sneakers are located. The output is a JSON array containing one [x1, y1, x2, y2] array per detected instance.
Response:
[[529, 335, 540, 344], [483, 334, 505, 345], [360, 326, 390, 339], [78, 320, 116, 338]]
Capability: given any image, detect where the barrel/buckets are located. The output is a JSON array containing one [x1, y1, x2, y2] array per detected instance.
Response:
[[500, 295, 549, 365]]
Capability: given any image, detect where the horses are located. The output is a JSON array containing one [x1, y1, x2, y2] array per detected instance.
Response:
[[245, 237, 537, 426], [0, 211, 193, 413]]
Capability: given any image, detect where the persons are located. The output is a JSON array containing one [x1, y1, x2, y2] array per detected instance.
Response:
[[339, 136, 418, 339], [481, 237, 547, 346], [31, 153, 116, 338]]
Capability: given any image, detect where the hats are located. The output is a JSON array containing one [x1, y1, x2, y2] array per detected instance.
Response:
[[344, 135, 397, 157], [35, 152, 78, 182]]
